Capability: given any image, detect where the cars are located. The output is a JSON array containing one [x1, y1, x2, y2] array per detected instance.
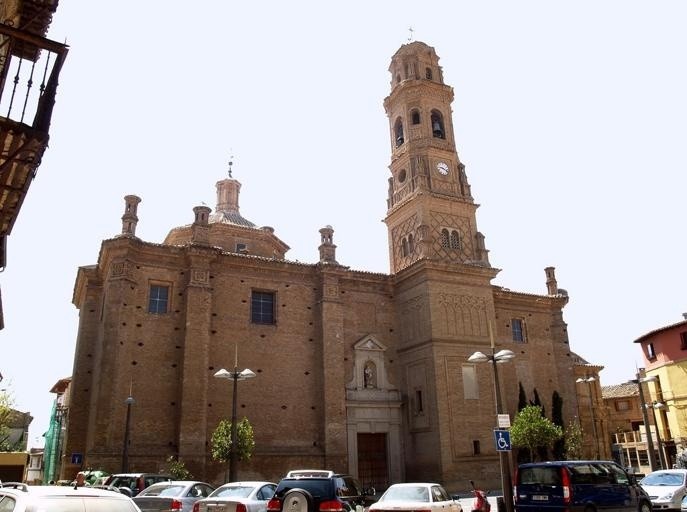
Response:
[[638, 469, 687, 512]]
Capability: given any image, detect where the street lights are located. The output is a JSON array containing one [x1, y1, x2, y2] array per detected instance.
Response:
[[575, 369, 601, 460], [466, 319, 517, 512], [640, 400, 668, 470], [213, 343, 256, 482], [621, 362, 658, 472], [121, 376, 136, 472]]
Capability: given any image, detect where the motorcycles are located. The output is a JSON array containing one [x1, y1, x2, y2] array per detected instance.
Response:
[[470, 479, 491, 512]]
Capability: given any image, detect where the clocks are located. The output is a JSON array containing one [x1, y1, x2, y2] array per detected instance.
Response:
[[436, 160, 449, 176]]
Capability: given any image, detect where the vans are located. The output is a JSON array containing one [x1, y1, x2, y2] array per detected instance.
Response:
[[513, 460, 653, 512]]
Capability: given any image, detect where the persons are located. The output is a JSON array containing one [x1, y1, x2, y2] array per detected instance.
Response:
[[69, 471, 90, 488], [137, 475, 150, 493]]
